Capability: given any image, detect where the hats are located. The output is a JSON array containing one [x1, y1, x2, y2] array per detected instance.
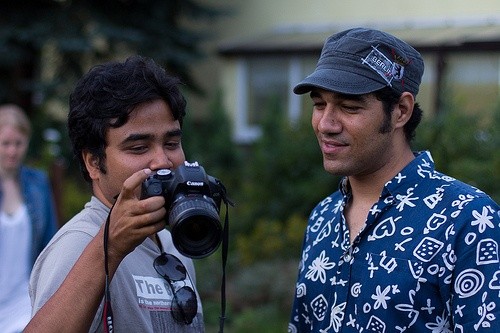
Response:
[[293, 27, 424, 100]]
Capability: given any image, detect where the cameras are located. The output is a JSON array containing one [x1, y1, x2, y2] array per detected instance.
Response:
[[143, 160, 230, 260]]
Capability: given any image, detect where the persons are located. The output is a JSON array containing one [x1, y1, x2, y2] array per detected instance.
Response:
[[20, 67, 205, 332], [0, 107, 55, 333], [288, 29, 500, 333]]
